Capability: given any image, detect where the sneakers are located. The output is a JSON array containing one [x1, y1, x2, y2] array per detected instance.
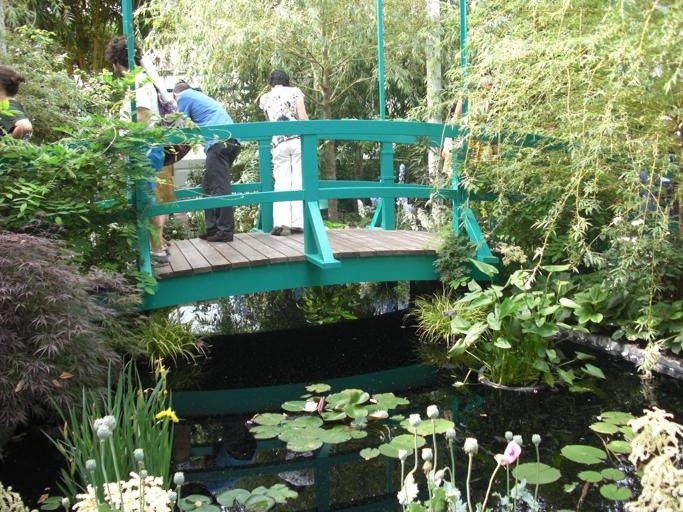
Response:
[[291, 227, 303, 234], [131, 238, 171, 271], [271, 227, 283, 235], [199, 233, 233, 242]]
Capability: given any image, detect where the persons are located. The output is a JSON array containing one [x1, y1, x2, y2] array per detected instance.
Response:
[[104, 32, 168, 269], [258, 68, 312, 235], [0, 62, 35, 144], [171, 79, 242, 243]]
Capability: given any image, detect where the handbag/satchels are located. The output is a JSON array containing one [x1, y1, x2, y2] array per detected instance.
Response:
[[160, 101, 202, 167]]
